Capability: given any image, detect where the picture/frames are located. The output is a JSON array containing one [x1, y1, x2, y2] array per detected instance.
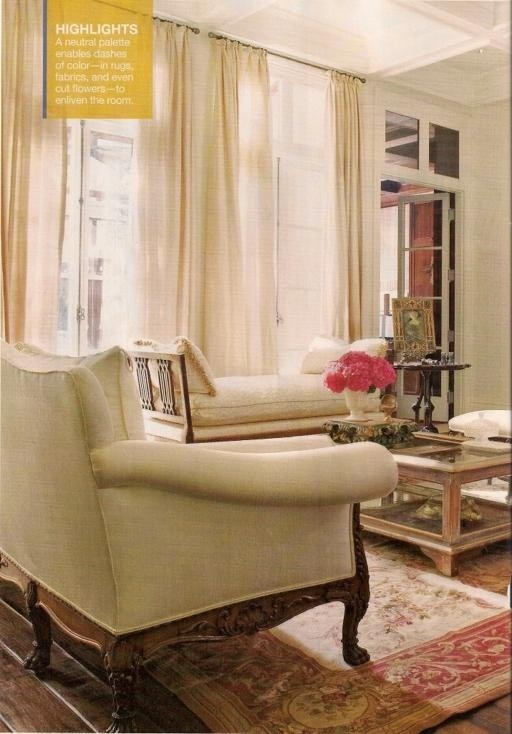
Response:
[[391, 297, 436, 363]]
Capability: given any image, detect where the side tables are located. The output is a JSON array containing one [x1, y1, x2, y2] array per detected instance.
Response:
[[393, 361, 470, 433]]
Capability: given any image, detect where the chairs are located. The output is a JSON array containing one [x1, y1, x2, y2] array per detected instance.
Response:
[[2, 340, 398, 733]]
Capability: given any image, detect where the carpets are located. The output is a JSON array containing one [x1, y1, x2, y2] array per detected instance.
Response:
[[141, 548, 511, 732]]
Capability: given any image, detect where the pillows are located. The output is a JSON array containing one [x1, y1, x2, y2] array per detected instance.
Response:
[[11, 342, 147, 442], [340, 338, 389, 361], [301, 336, 350, 375], [130, 337, 215, 395]]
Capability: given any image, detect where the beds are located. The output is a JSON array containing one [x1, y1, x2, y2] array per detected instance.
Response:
[[120, 334, 396, 446]]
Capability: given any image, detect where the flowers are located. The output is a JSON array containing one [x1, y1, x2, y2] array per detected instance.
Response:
[[323, 351, 397, 393]]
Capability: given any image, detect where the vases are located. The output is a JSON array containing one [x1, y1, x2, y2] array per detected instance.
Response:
[[343, 385, 373, 422]]
